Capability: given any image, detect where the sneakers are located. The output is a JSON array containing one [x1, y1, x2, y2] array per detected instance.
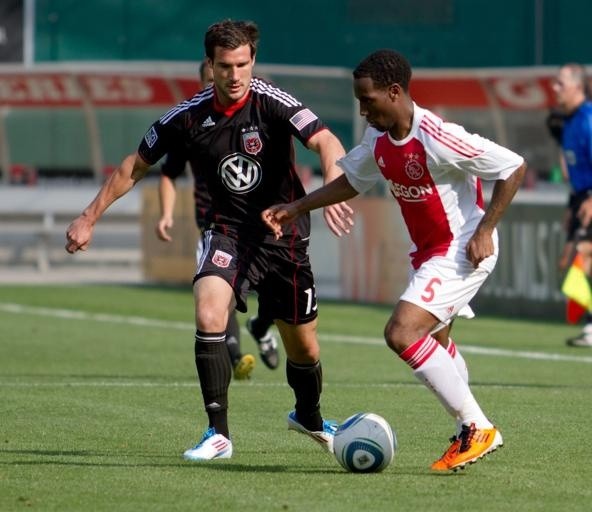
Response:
[[287, 410, 335, 453], [232, 355, 255, 380], [184, 427, 233, 460], [432, 424, 504, 472], [567, 323, 592, 347], [247, 315, 279, 368]]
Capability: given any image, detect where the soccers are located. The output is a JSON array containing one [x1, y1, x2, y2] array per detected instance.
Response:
[[333, 412, 394, 473]]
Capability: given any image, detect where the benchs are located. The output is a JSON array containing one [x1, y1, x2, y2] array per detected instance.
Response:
[[0, 184, 145, 282]]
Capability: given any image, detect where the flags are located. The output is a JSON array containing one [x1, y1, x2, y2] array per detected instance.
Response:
[[560, 253, 592, 323]]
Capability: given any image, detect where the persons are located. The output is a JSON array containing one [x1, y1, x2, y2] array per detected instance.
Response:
[[64, 19, 356, 465], [152, 58, 281, 383], [262, 52, 529, 472], [548, 61, 592, 349]]
